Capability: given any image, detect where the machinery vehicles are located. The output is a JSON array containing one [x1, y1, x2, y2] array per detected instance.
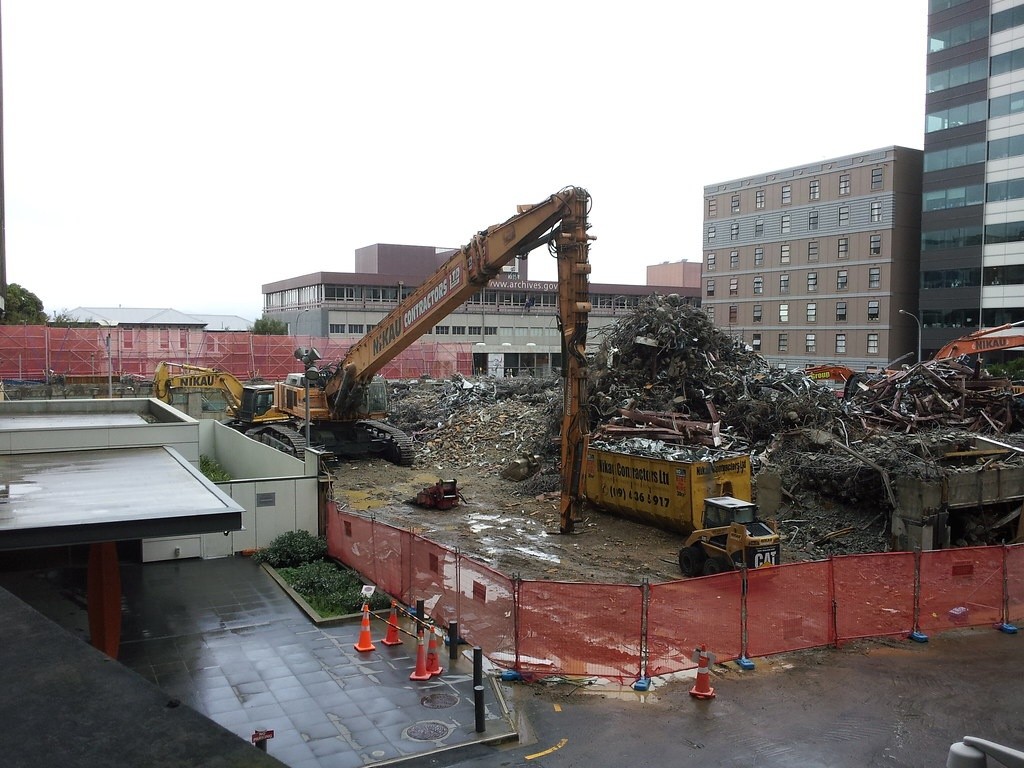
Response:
[[244, 187, 591, 537], [154, 361, 291, 429], [678, 496, 782, 575]]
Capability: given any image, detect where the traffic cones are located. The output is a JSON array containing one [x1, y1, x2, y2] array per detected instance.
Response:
[[688, 642, 716, 700], [424, 625, 443, 675], [354, 605, 375, 653], [380, 601, 403, 647], [409, 630, 431, 680]]
